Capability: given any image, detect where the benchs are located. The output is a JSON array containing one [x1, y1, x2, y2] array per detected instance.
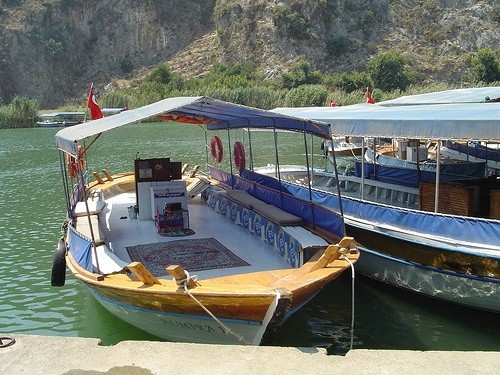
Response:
[[201, 184, 329, 269], [71, 188, 129, 276]]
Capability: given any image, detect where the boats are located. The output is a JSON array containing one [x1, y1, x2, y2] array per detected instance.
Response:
[[237, 86, 500, 319], [36, 112, 87, 127], [54, 96, 361, 345]]
